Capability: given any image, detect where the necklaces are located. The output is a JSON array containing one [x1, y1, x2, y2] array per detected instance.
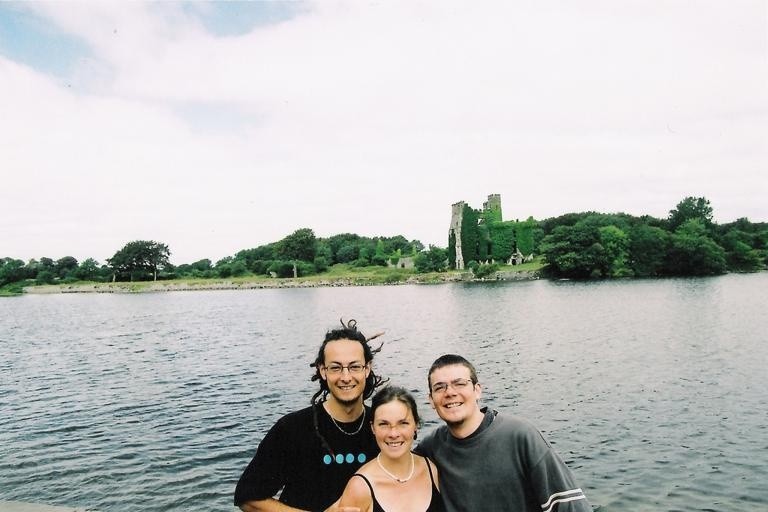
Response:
[[324, 401, 367, 437], [376, 451, 415, 483]]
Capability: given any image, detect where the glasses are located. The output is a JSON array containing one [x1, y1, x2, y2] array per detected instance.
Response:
[[323, 363, 367, 374], [429, 377, 475, 395]]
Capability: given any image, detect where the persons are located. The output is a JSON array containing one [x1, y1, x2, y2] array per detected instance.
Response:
[[336, 384, 448, 512], [406, 353, 599, 512], [232, 320, 382, 512]]
